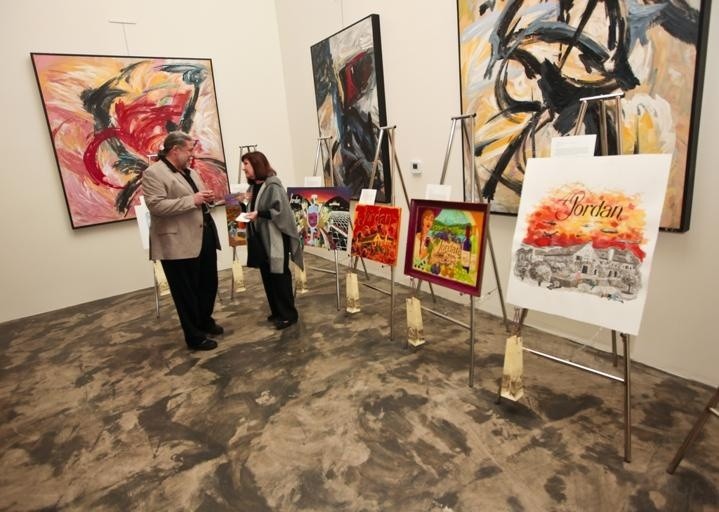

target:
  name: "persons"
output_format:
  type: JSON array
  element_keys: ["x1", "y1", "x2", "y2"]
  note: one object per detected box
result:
[
  {"x1": 140, "y1": 131, "x2": 224, "y2": 352},
  {"x1": 234, "y1": 151, "x2": 309, "y2": 336},
  {"x1": 410, "y1": 209, "x2": 435, "y2": 272}
]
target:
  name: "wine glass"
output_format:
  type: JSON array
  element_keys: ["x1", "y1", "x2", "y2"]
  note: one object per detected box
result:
[{"x1": 306, "y1": 206, "x2": 319, "y2": 245}]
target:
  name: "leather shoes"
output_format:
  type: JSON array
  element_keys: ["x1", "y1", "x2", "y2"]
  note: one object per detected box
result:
[
  {"x1": 187, "y1": 338, "x2": 218, "y2": 350},
  {"x1": 207, "y1": 322, "x2": 224, "y2": 334},
  {"x1": 267, "y1": 314, "x2": 298, "y2": 329}
]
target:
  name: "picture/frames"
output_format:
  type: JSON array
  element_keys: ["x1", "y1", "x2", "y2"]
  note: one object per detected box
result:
[
  {"x1": 30, "y1": 52, "x2": 230, "y2": 230},
  {"x1": 455, "y1": 0, "x2": 713, "y2": 233},
  {"x1": 404, "y1": 199, "x2": 491, "y2": 298},
  {"x1": 309, "y1": 14, "x2": 391, "y2": 204}
]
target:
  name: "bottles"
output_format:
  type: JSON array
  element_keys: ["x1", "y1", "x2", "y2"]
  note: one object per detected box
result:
[
  {"x1": 230, "y1": 224, "x2": 236, "y2": 237},
  {"x1": 470, "y1": 225, "x2": 477, "y2": 269},
  {"x1": 461, "y1": 225, "x2": 470, "y2": 271}
]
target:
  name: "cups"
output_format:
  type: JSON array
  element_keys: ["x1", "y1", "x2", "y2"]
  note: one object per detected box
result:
[{"x1": 237, "y1": 229, "x2": 247, "y2": 238}]
